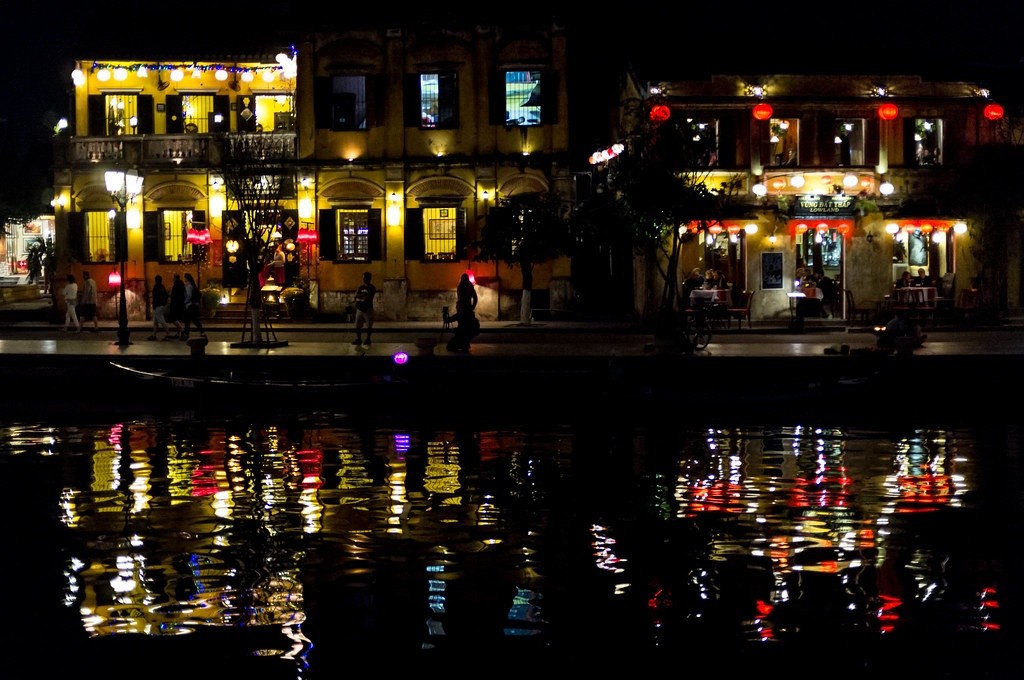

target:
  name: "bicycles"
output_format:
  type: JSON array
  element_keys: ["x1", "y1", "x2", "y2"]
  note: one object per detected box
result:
[{"x1": 683, "y1": 302, "x2": 722, "y2": 356}]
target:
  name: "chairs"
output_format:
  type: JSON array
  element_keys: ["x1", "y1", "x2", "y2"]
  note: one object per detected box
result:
[
  {"x1": 845, "y1": 288, "x2": 871, "y2": 327},
  {"x1": 912, "y1": 290, "x2": 937, "y2": 328},
  {"x1": 934, "y1": 273, "x2": 954, "y2": 308},
  {"x1": 955, "y1": 288, "x2": 979, "y2": 326},
  {"x1": 891, "y1": 289, "x2": 910, "y2": 323},
  {"x1": 728, "y1": 290, "x2": 756, "y2": 329}
]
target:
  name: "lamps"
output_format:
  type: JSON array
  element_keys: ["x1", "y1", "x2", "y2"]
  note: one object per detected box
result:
[
  {"x1": 391, "y1": 193, "x2": 397, "y2": 200},
  {"x1": 834, "y1": 124, "x2": 843, "y2": 144},
  {"x1": 866, "y1": 230, "x2": 874, "y2": 243},
  {"x1": 129, "y1": 116, "x2": 140, "y2": 129},
  {"x1": 483, "y1": 190, "x2": 488, "y2": 198},
  {"x1": 94, "y1": 68, "x2": 111, "y2": 84},
  {"x1": 58, "y1": 194, "x2": 67, "y2": 208},
  {"x1": 769, "y1": 119, "x2": 780, "y2": 144},
  {"x1": 299, "y1": 177, "x2": 311, "y2": 189},
  {"x1": 780, "y1": 120, "x2": 790, "y2": 129},
  {"x1": 210, "y1": 180, "x2": 220, "y2": 190},
  {"x1": 764, "y1": 222, "x2": 780, "y2": 245},
  {"x1": 843, "y1": 119, "x2": 854, "y2": 132}
]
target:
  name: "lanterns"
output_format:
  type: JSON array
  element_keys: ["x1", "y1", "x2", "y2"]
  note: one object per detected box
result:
[
  {"x1": 750, "y1": 105, "x2": 775, "y2": 120},
  {"x1": 649, "y1": 103, "x2": 673, "y2": 121},
  {"x1": 875, "y1": 101, "x2": 900, "y2": 122},
  {"x1": 983, "y1": 102, "x2": 1005, "y2": 121}
]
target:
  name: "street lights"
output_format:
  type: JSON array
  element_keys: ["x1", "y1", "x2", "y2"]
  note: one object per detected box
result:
[{"x1": 105, "y1": 163, "x2": 145, "y2": 347}]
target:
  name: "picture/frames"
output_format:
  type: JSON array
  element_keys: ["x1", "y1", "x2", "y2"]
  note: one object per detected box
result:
[
  {"x1": 231, "y1": 103, "x2": 237, "y2": 111},
  {"x1": 760, "y1": 250, "x2": 786, "y2": 292},
  {"x1": 156, "y1": 104, "x2": 165, "y2": 112}
]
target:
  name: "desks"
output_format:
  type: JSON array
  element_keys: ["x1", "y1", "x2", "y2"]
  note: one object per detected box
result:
[
  {"x1": 794, "y1": 288, "x2": 823, "y2": 299},
  {"x1": 892, "y1": 285, "x2": 938, "y2": 305},
  {"x1": 689, "y1": 288, "x2": 731, "y2": 311}
]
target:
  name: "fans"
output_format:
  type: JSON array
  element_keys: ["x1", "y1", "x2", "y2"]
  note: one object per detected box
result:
[
  {"x1": 227, "y1": 62, "x2": 241, "y2": 92},
  {"x1": 156, "y1": 61, "x2": 170, "y2": 92}
]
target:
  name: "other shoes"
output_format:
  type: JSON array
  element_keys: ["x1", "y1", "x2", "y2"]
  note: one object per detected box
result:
[
  {"x1": 146, "y1": 335, "x2": 156, "y2": 341},
  {"x1": 161, "y1": 337, "x2": 170, "y2": 341},
  {"x1": 364, "y1": 337, "x2": 372, "y2": 345},
  {"x1": 200, "y1": 334, "x2": 207, "y2": 338},
  {"x1": 352, "y1": 338, "x2": 363, "y2": 345},
  {"x1": 179, "y1": 335, "x2": 189, "y2": 341},
  {"x1": 170, "y1": 334, "x2": 180, "y2": 339},
  {"x1": 59, "y1": 328, "x2": 67, "y2": 333}
]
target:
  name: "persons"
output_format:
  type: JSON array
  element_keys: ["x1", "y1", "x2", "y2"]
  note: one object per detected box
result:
[
  {"x1": 351, "y1": 272, "x2": 375, "y2": 344},
  {"x1": 894, "y1": 271, "x2": 913, "y2": 286},
  {"x1": 58, "y1": 274, "x2": 81, "y2": 335},
  {"x1": 182, "y1": 273, "x2": 207, "y2": 338},
  {"x1": 442, "y1": 302, "x2": 479, "y2": 351},
  {"x1": 77, "y1": 271, "x2": 99, "y2": 333},
  {"x1": 688, "y1": 264, "x2": 745, "y2": 318},
  {"x1": 165, "y1": 273, "x2": 186, "y2": 337},
  {"x1": 146, "y1": 275, "x2": 170, "y2": 341},
  {"x1": 922, "y1": 149, "x2": 935, "y2": 165},
  {"x1": 456, "y1": 273, "x2": 478, "y2": 311},
  {"x1": 792, "y1": 257, "x2": 839, "y2": 318},
  {"x1": 915, "y1": 267, "x2": 932, "y2": 284}
]
